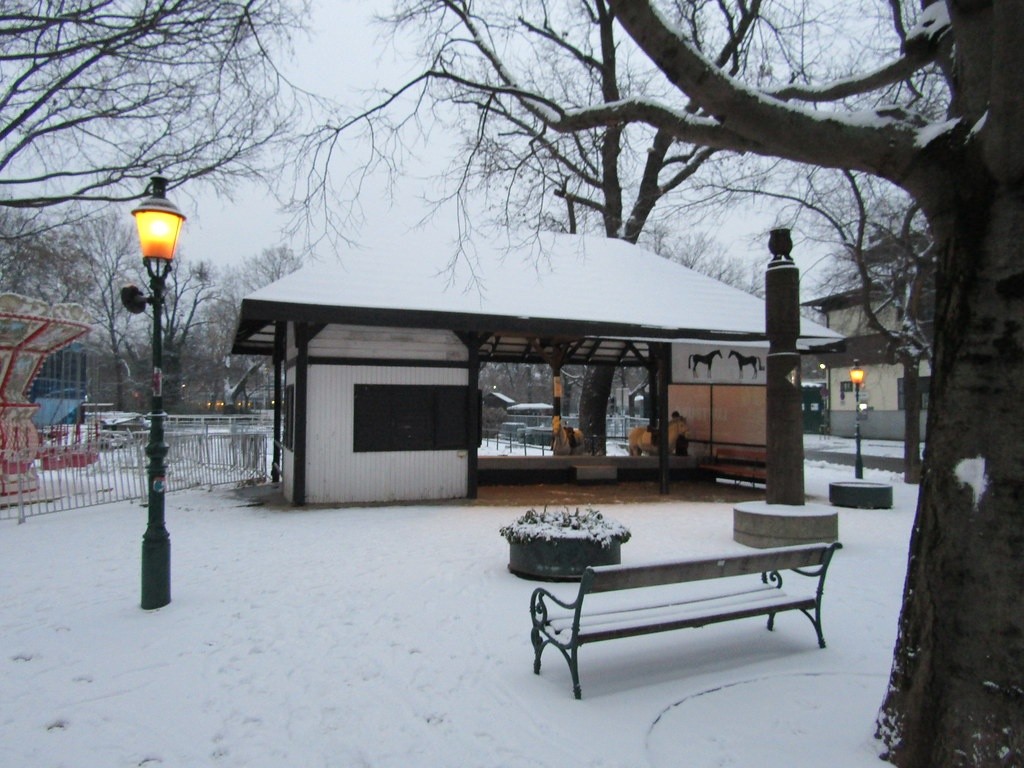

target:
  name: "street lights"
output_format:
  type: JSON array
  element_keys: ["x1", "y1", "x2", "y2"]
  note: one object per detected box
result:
[
  {"x1": 848, "y1": 359, "x2": 873, "y2": 481},
  {"x1": 118, "y1": 161, "x2": 186, "y2": 609}
]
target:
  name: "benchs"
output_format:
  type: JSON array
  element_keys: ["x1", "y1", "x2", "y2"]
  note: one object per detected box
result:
[{"x1": 528, "y1": 542, "x2": 844, "y2": 700}]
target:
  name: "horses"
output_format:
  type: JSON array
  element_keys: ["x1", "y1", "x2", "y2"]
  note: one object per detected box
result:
[
  {"x1": 551, "y1": 415, "x2": 584, "y2": 456},
  {"x1": 629, "y1": 416, "x2": 691, "y2": 457}
]
